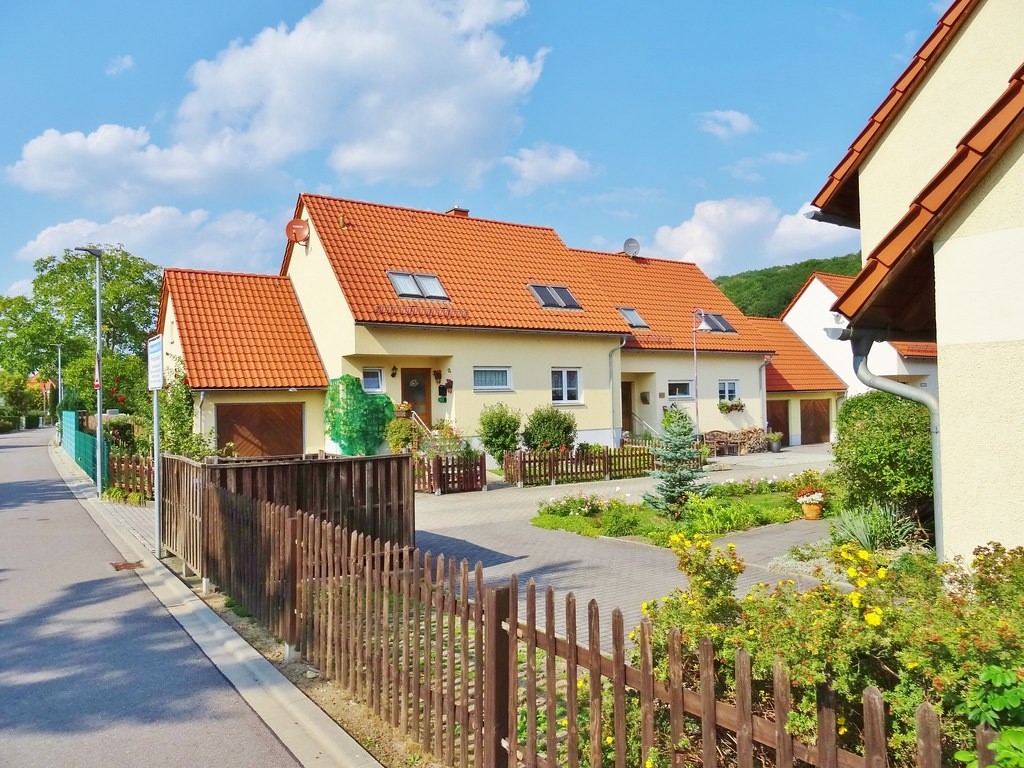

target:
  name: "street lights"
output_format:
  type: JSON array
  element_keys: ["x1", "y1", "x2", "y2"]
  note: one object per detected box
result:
[
  {"x1": 692, "y1": 307, "x2": 713, "y2": 443},
  {"x1": 49, "y1": 343, "x2": 64, "y2": 448},
  {"x1": 74, "y1": 247, "x2": 103, "y2": 499}
]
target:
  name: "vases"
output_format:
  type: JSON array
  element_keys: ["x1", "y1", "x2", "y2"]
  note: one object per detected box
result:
[
  {"x1": 448, "y1": 388, "x2": 452, "y2": 393},
  {"x1": 393, "y1": 410, "x2": 411, "y2": 417},
  {"x1": 802, "y1": 503, "x2": 822, "y2": 520},
  {"x1": 436, "y1": 378, "x2": 440, "y2": 383}
]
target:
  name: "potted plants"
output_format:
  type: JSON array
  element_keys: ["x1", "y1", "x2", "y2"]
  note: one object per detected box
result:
[
  {"x1": 766, "y1": 432, "x2": 784, "y2": 453},
  {"x1": 717, "y1": 401, "x2": 731, "y2": 414},
  {"x1": 732, "y1": 399, "x2": 746, "y2": 412}
]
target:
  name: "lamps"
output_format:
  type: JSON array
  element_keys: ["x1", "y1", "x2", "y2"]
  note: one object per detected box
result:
[{"x1": 391, "y1": 365, "x2": 398, "y2": 377}]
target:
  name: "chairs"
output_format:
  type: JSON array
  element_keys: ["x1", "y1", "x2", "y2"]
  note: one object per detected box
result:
[{"x1": 703, "y1": 430, "x2": 741, "y2": 458}]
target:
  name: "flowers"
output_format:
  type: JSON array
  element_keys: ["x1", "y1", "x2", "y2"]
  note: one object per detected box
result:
[
  {"x1": 795, "y1": 485, "x2": 832, "y2": 504},
  {"x1": 446, "y1": 379, "x2": 454, "y2": 388},
  {"x1": 433, "y1": 369, "x2": 441, "y2": 379},
  {"x1": 394, "y1": 401, "x2": 412, "y2": 410}
]
